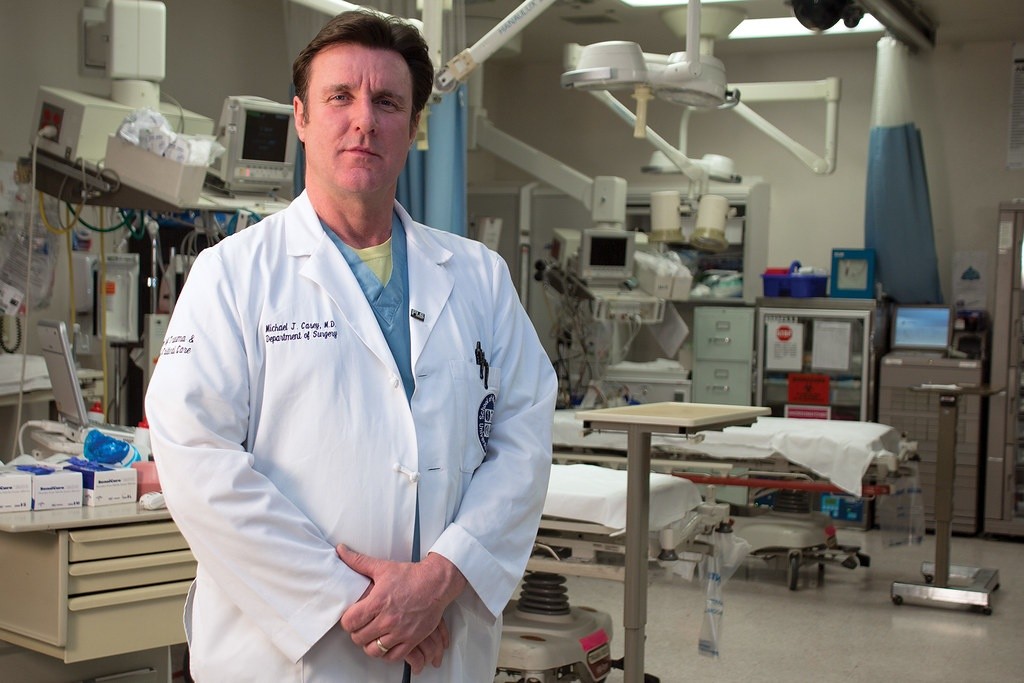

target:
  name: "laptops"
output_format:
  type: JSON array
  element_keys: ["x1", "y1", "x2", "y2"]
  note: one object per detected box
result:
[
  {"x1": 34, "y1": 318, "x2": 138, "y2": 440},
  {"x1": 884, "y1": 304, "x2": 954, "y2": 361}
]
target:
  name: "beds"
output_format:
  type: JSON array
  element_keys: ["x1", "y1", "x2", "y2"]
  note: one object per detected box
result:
[
  {"x1": 524, "y1": 463, "x2": 730, "y2": 586},
  {"x1": 553, "y1": 408, "x2": 920, "y2": 590}
]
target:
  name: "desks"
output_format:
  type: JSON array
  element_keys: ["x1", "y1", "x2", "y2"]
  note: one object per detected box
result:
[
  {"x1": 888, "y1": 382, "x2": 1006, "y2": 615},
  {"x1": 573, "y1": 398, "x2": 770, "y2": 683}
]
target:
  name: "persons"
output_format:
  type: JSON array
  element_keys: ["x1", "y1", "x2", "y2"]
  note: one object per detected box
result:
[{"x1": 145, "y1": 12, "x2": 559, "y2": 683}]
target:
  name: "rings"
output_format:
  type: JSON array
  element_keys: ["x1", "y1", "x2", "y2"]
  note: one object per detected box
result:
[{"x1": 375, "y1": 637, "x2": 389, "y2": 653}]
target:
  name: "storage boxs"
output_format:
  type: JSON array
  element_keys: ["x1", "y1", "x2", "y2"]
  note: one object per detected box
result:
[
  {"x1": 0, "y1": 457, "x2": 137, "y2": 514},
  {"x1": 761, "y1": 261, "x2": 831, "y2": 298},
  {"x1": 103, "y1": 134, "x2": 205, "y2": 209}
]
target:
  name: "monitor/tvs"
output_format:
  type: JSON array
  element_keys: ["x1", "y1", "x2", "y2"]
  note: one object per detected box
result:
[
  {"x1": 579, "y1": 229, "x2": 635, "y2": 280},
  {"x1": 209, "y1": 96, "x2": 298, "y2": 181}
]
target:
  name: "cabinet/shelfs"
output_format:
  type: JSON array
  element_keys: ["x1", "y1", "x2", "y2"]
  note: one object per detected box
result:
[{"x1": 690, "y1": 304, "x2": 756, "y2": 404}]
